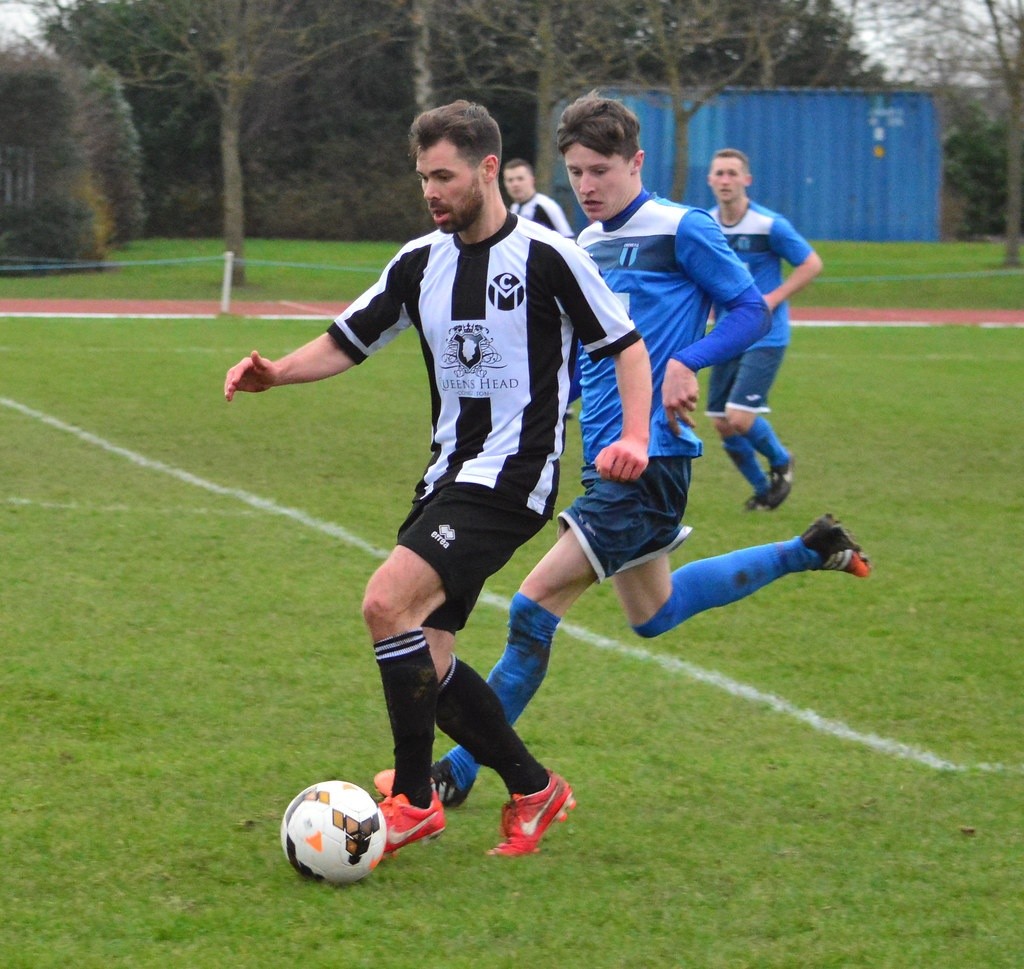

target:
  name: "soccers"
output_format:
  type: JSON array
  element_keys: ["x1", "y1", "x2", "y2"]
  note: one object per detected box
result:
[{"x1": 280, "y1": 779, "x2": 386, "y2": 885}]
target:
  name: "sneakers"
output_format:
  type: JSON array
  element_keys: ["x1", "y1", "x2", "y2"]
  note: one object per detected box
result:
[
  {"x1": 377, "y1": 777, "x2": 446, "y2": 853},
  {"x1": 766, "y1": 452, "x2": 795, "y2": 508},
  {"x1": 373, "y1": 759, "x2": 477, "y2": 808},
  {"x1": 746, "y1": 493, "x2": 773, "y2": 510},
  {"x1": 800, "y1": 513, "x2": 872, "y2": 577},
  {"x1": 485, "y1": 768, "x2": 577, "y2": 857}
]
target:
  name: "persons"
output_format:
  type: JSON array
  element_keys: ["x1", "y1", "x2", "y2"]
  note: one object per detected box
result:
[
  {"x1": 502, "y1": 159, "x2": 576, "y2": 239},
  {"x1": 220, "y1": 99, "x2": 651, "y2": 856},
  {"x1": 703, "y1": 148, "x2": 825, "y2": 511},
  {"x1": 374, "y1": 90, "x2": 869, "y2": 806}
]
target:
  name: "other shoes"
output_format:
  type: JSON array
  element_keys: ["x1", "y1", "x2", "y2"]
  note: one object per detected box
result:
[{"x1": 566, "y1": 406, "x2": 574, "y2": 418}]
